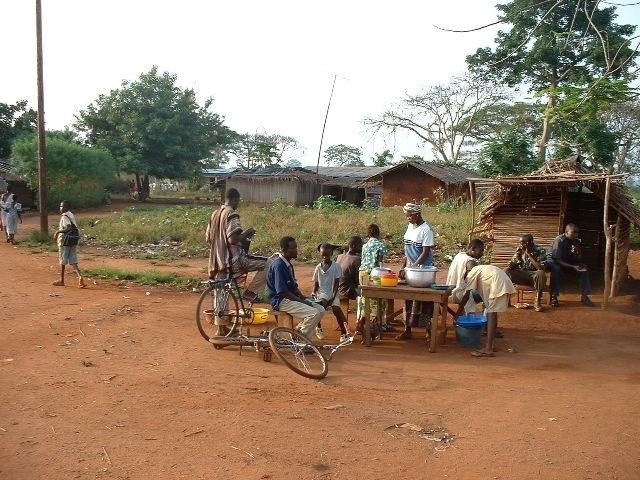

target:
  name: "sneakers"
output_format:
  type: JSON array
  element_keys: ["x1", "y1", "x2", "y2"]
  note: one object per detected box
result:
[
  {"x1": 382, "y1": 324, "x2": 392, "y2": 332},
  {"x1": 316, "y1": 327, "x2": 324, "y2": 340},
  {"x1": 340, "y1": 334, "x2": 350, "y2": 343},
  {"x1": 534, "y1": 302, "x2": 543, "y2": 312}
]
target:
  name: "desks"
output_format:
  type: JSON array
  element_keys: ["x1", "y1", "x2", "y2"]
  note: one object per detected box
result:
[{"x1": 357, "y1": 283, "x2": 449, "y2": 354}]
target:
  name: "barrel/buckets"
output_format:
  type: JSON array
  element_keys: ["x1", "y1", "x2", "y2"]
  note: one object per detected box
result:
[{"x1": 456, "y1": 325, "x2": 482, "y2": 346}]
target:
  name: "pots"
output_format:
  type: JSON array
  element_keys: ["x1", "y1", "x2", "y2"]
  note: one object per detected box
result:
[{"x1": 402, "y1": 263, "x2": 437, "y2": 287}]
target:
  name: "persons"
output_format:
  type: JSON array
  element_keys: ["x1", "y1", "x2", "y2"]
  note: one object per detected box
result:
[
  {"x1": 0, "y1": 185, "x2": 12, "y2": 236},
  {"x1": 454, "y1": 261, "x2": 517, "y2": 357},
  {"x1": 310, "y1": 244, "x2": 353, "y2": 344},
  {"x1": 0, "y1": 193, "x2": 22, "y2": 243},
  {"x1": 354, "y1": 223, "x2": 385, "y2": 338},
  {"x1": 335, "y1": 236, "x2": 395, "y2": 332},
  {"x1": 394, "y1": 203, "x2": 436, "y2": 340},
  {"x1": 51, "y1": 200, "x2": 85, "y2": 288},
  {"x1": 546, "y1": 223, "x2": 594, "y2": 306},
  {"x1": 502, "y1": 234, "x2": 548, "y2": 311},
  {"x1": 130, "y1": 179, "x2": 136, "y2": 200},
  {"x1": 206, "y1": 188, "x2": 268, "y2": 337},
  {"x1": 265, "y1": 235, "x2": 325, "y2": 355},
  {"x1": 447, "y1": 239, "x2": 505, "y2": 337}
]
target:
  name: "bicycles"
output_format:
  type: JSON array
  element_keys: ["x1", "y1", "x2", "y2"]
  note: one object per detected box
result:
[
  {"x1": 196, "y1": 255, "x2": 270, "y2": 342},
  {"x1": 208, "y1": 326, "x2": 358, "y2": 380}
]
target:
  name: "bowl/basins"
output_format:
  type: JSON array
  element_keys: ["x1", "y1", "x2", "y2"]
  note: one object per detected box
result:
[
  {"x1": 242, "y1": 308, "x2": 270, "y2": 325},
  {"x1": 374, "y1": 274, "x2": 399, "y2": 286},
  {"x1": 457, "y1": 315, "x2": 488, "y2": 329},
  {"x1": 370, "y1": 267, "x2": 392, "y2": 279},
  {"x1": 203, "y1": 308, "x2": 234, "y2": 325}
]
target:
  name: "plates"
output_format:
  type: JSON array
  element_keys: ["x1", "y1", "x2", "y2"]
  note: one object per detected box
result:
[{"x1": 431, "y1": 284, "x2": 450, "y2": 289}]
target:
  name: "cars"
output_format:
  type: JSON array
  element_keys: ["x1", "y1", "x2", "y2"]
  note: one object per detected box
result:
[{"x1": 148, "y1": 181, "x2": 174, "y2": 192}]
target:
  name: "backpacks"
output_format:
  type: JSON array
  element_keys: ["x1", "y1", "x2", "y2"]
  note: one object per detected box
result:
[{"x1": 61, "y1": 215, "x2": 81, "y2": 246}]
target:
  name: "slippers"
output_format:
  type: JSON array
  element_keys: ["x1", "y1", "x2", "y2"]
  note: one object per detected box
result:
[
  {"x1": 395, "y1": 331, "x2": 413, "y2": 341},
  {"x1": 80, "y1": 284, "x2": 87, "y2": 288},
  {"x1": 471, "y1": 349, "x2": 495, "y2": 357},
  {"x1": 424, "y1": 333, "x2": 431, "y2": 342},
  {"x1": 243, "y1": 294, "x2": 263, "y2": 302},
  {"x1": 53, "y1": 281, "x2": 65, "y2": 286}
]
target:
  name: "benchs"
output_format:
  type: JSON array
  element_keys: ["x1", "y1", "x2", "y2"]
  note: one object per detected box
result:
[
  {"x1": 384, "y1": 295, "x2": 465, "y2": 330},
  {"x1": 506, "y1": 270, "x2": 554, "y2": 308},
  {"x1": 268, "y1": 293, "x2": 351, "y2": 343}
]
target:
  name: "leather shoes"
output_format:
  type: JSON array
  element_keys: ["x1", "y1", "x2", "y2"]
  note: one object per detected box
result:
[
  {"x1": 550, "y1": 294, "x2": 559, "y2": 307},
  {"x1": 581, "y1": 295, "x2": 593, "y2": 306}
]
